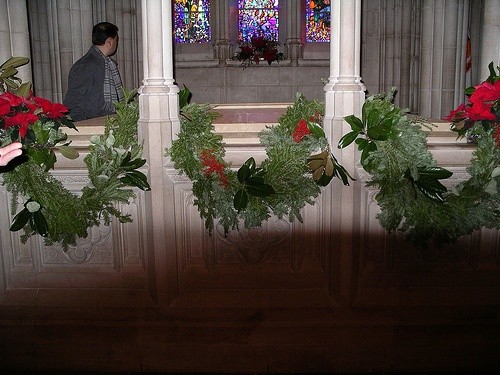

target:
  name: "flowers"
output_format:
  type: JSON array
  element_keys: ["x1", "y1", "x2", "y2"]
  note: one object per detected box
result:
[
  {"x1": 234, "y1": 37, "x2": 286, "y2": 70},
  {"x1": 0, "y1": 61, "x2": 500, "y2": 252}
]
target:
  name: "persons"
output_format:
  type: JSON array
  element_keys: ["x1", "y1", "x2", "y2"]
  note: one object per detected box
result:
[{"x1": 65, "y1": 22, "x2": 126, "y2": 122}]
